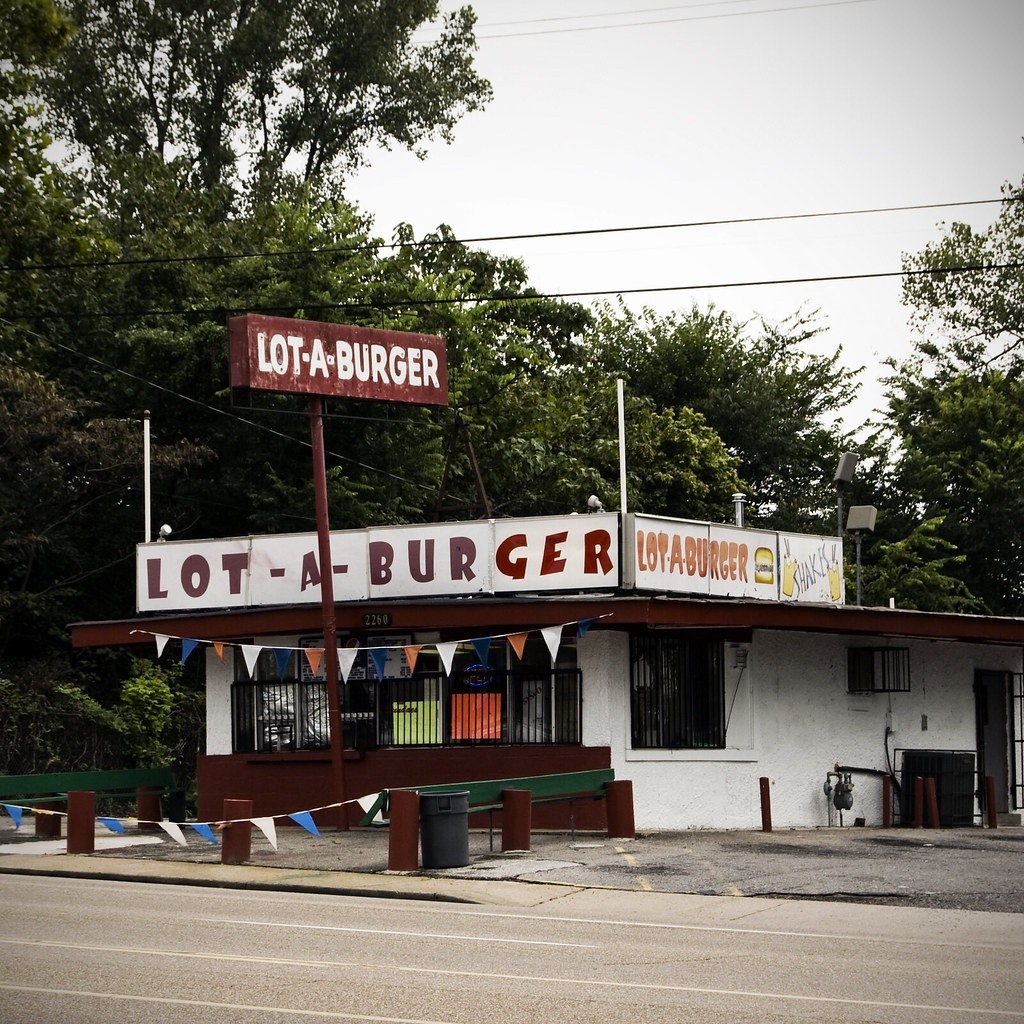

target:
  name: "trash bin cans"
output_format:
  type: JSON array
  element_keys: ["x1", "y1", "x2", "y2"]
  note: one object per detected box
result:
[
  {"x1": 419, "y1": 789, "x2": 472, "y2": 871},
  {"x1": 901, "y1": 751, "x2": 976, "y2": 827}
]
